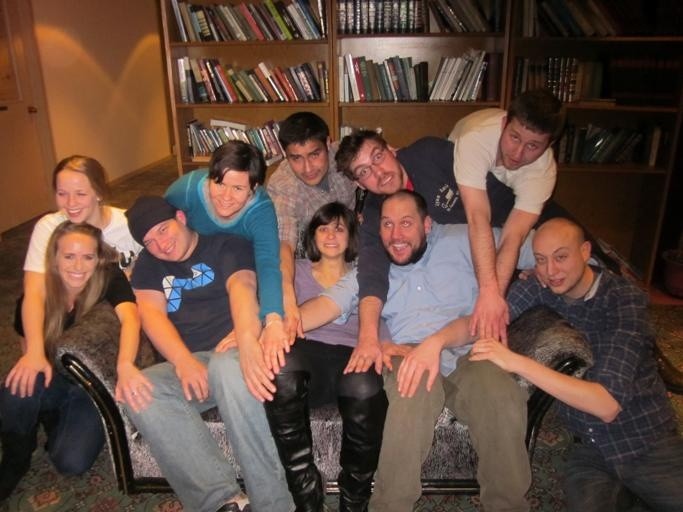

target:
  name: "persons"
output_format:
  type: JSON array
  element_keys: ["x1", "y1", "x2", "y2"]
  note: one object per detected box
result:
[
  {"x1": 162, "y1": 140, "x2": 289, "y2": 373},
  {"x1": 396, "y1": 218, "x2": 682, "y2": 512},
  {"x1": 334, "y1": 129, "x2": 682, "y2": 396},
  {"x1": 447, "y1": 90, "x2": 559, "y2": 347},
  {"x1": 123, "y1": 196, "x2": 295, "y2": 511},
  {"x1": 217, "y1": 190, "x2": 604, "y2": 511},
  {"x1": 6, "y1": 155, "x2": 142, "y2": 397},
  {"x1": 215, "y1": 202, "x2": 412, "y2": 511},
  {"x1": 268, "y1": 112, "x2": 359, "y2": 346},
  {"x1": 0, "y1": 219, "x2": 153, "y2": 503}
]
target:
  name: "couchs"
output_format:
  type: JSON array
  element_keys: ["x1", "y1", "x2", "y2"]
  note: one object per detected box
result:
[{"x1": 55, "y1": 297, "x2": 594, "y2": 493}]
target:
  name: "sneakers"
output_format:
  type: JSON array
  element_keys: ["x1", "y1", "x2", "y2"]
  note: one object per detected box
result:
[{"x1": 0, "y1": 461, "x2": 30, "y2": 500}]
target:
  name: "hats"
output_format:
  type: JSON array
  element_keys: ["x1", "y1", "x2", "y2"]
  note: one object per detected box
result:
[{"x1": 124, "y1": 195, "x2": 180, "y2": 247}]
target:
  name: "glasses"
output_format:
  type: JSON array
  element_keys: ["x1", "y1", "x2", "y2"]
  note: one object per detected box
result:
[{"x1": 352, "y1": 149, "x2": 386, "y2": 180}]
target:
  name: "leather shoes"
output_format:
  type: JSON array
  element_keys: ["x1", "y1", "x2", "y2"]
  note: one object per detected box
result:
[
  {"x1": 660, "y1": 356, "x2": 683, "y2": 394},
  {"x1": 215, "y1": 502, "x2": 252, "y2": 512}
]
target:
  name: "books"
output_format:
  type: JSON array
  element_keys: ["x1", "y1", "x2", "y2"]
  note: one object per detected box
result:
[
  {"x1": 556, "y1": 110, "x2": 672, "y2": 168},
  {"x1": 338, "y1": 1, "x2": 507, "y2": 35},
  {"x1": 523, "y1": 0, "x2": 619, "y2": 38},
  {"x1": 177, "y1": 55, "x2": 327, "y2": 103},
  {"x1": 338, "y1": 47, "x2": 500, "y2": 102},
  {"x1": 185, "y1": 118, "x2": 286, "y2": 160},
  {"x1": 597, "y1": 235, "x2": 638, "y2": 278},
  {"x1": 173, "y1": 0, "x2": 327, "y2": 43},
  {"x1": 515, "y1": 54, "x2": 683, "y2": 106},
  {"x1": 339, "y1": 124, "x2": 382, "y2": 140}
]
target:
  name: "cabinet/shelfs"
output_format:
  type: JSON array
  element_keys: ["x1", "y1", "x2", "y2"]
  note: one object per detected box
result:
[
  {"x1": 156, "y1": 1, "x2": 332, "y2": 175},
  {"x1": 505, "y1": 2, "x2": 683, "y2": 288},
  {"x1": 330, "y1": 1, "x2": 504, "y2": 144}
]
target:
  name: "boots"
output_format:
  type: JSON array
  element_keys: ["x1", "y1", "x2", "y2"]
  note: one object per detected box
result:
[
  {"x1": 263, "y1": 369, "x2": 324, "y2": 512},
  {"x1": 337, "y1": 390, "x2": 389, "y2": 512}
]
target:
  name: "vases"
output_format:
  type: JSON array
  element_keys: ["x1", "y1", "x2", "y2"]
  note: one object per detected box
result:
[{"x1": 663, "y1": 249, "x2": 683, "y2": 298}]
